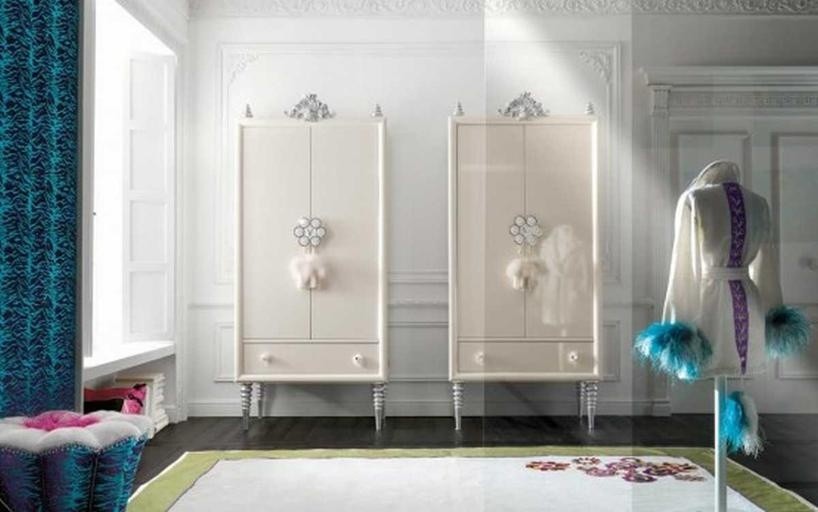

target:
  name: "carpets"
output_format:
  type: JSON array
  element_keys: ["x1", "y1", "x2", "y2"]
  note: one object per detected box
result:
[{"x1": 119, "y1": 446, "x2": 818, "y2": 511}]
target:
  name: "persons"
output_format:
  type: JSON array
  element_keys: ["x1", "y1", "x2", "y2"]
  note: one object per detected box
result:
[
  {"x1": 533, "y1": 223, "x2": 591, "y2": 332},
  {"x1": 629, "y1": 158, "x2": 811, "y2": 381}
]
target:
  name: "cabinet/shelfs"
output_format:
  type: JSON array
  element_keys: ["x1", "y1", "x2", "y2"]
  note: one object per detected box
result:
[
  {"x1": 235, "y1": 117, "x2": 388, "y2": 431},
  {"x1": 446, "y1": 115, "x2": 601, "y2": 430}
]
